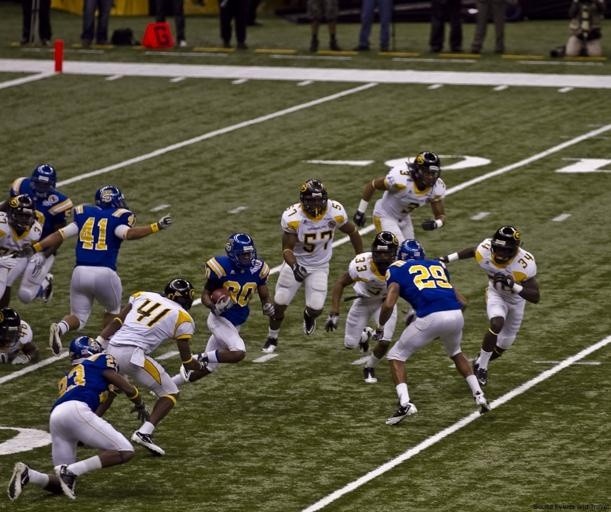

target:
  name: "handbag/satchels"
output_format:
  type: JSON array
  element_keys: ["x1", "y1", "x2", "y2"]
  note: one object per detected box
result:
[{"x1": 111, "y1": 28, "x2": 135, "y2": 47}]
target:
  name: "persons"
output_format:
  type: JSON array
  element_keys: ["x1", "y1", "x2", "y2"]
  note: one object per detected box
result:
[
  {"x1": 21, "y1": 183, "x2": 174, "y2": 353},
  {"x1": 372, "y1": 239, "x2": 492, "y2": 427},
  {"x1": 77, "y1": 276, "x2": 215, "y2": 458},
  {"x1": 16, "y1": 1, "x2": 55, "y2": 49},
  {"x1": 304, "y1": 0, "x2": 344, "y2": 53},
  {"x1": 434, "y1": 224, "x2": 543, "y2": 385},
  {"x1": 354, "y1": 152, "x2": 449, "y2": 255},
  {"x1": 1, "y1": 195, "x2": 46, "y2": 301},
  {"x1": 429, "y1": 1, "x2": 466, "y2": 56},
  {"x1": 80, "y1": 0, "x2": 114, "y2": 50},
  {"x1": 565, "y1": 0, "x2": 609, "y2": 58},
  {"x1": 217, "y1": 0, "x2": 250, "y2": 51},
  {"x1": 324, "y1": 233, "x2": 403, "y2": 382},
  {"x1": 7, "y1": 335, "x2": 152, "y2": 502},
  {"x1": 0, "y1": 305, "x2": 40, "y2": 367},
  {"x1": 155, "y1": 0, "x2": 188, "y2": 49},
  {"x1": 469, "y1": 0, "x2": 514, "y2": 55},
  {"x1": 351, "y1": 0, "x2": 397, "y2": 54},
  {"x1": 171, "y1": 233, "x2": 276, "y2": 386},
  {"x1": 1, "y1": 165, "x2": 75, "y2": 309},
  {"x1": 260, "y1": 180, "x2": 366, "y2": 354}
]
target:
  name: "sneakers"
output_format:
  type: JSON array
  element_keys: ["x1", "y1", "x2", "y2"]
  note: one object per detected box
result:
[
  {"x1": 356, "y1": 326, "x2": 371, "y2": 352},
  {"x1": 7, "y1": 461, "x2": 34, "y2": 502},
  {"x1": 131, "y1": 428, "x2": 166, "y2": 456},
  {"x1": 260, "y1": 338, "x2": 278, "y2": 353},
  {"x1": 473, "y1": 360, "x2": 488, "y2": 388},
  {"x1": 302, "y1": 308, "x2": 316, "y2": 335},
  {"x1": 39, "y1": 275, "x2": 55, "y2": 302},
  {"x1": 475, "y1": 390, "x2": 491, "y2": 415},
  {"x1": 54, "y1": 463, "x2": 78, "y2": 501},
  {"x1": 180, "y1": 352, "x2": 199, "y2": 380},
  {"x1": 385, "y1": 401, "x2": 417, "y2": 425},
  {"x1": 49, "y1": 323, "x2": 63, "y2": 358},
  {"x1": 363, "y1": 362, "x2": 377, "y2": 386}
]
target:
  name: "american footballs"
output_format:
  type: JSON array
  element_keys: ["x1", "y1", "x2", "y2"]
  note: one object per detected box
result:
[{"x1": 210, "y1": 288, "x2": 230, "y2": 303}]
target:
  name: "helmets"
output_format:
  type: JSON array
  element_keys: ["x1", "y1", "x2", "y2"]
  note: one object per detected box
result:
[
  {"x1": 300, "y1": 179, "x2": 327, "y2": 218},
  {"x1": 397, "y1": 240, "x2": 427, "y2": 261},
  {"x1": 68, "y1": 336, "x2": 101, "y2": 365},
  {"x1": 491, "y1": 225, "x2": 521, "y2": 263},
  {"x1": 371, "y1": 230, "x2": 398, "y2": 276},
  {"x1": 162, "y1": 278, "x2": 196, "y2": 312},
  {"x1": 411, "y1": 151, "x2": 441, "y2": 190},
  {"x1": 0, "y1": 307, "x2": 20, "y2": 349},
  {"x1": 95, "y1": 186, "x2": 129, "y2": 210},
  {"x1": 7, "y1": 195, "x2": 38, "y2": 239},
  {"x1": 225, "y1": 233, "x2": 256, "y2": 273},
  {"x1": 30, "y1": 165, "x2": 57, "y2": 197}
]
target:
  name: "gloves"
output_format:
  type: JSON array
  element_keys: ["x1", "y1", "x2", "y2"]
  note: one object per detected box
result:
[
  {"x1": 325, "y1": 312, "x2": 340, "y2": 332},
  {"x1": 12, "y1": 354, "x2": 32, "y2": 364},
  {"x1": 14, "y1": 243, "x2": 41, "y2": 259},
  {"x1": 130, "y1": 403, "x2": 150, "y2": 425},
  {"x1": 293, "y1": 263, "x2": 310, "y2": 281},
  {"x1": 371, "y1": 328, "x2": 384, "y2": 340},
  {"x1": 199, "y1": 355, "x2": 209, "y2": 371},
  {"x1": 263, "y1": 302, "x2": 275, "y2": 315},
  {"x1": 421, "y1": 218, "x2": 439, "y2": 232},
  {"x1": 493, "y1": 272, "x2": 523, "y2": 294},
  {"x1": 353, "y1": 211, "x2": 366, "y2": 225},
  {"x1": 27, "y1": 253, "x2": 46, "y2": 276},
  {"x1": 0, "y1": 255, "x2": 16, "y2": 268},
  {"x1": 151, "y1": 212, "x2": 173, "y2": 233},
  {"x1": 216, "y1": 297, "x2": 232, "y2": 314}
]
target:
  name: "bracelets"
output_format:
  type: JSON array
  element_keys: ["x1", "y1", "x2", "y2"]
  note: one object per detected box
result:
[
  {"x1": 511, "y1": 282, "x2": 527, "y2": 295},
  {"x1": 434, "y1": 218, "x2": 445, "y2": 229},
  {"x1": 149, "y1": 222, "x2": 160, "y2": 233},
  {"x1": 358, "y1": 198, "x2": 369, "y2": 215},
  {"x1": 446, "y1": 251, "x2": 462, "y2": 262},
  {"x1": 31, "y1": 240, "x2": 43, "y2": 254},
  {"x1": 134, "y1": 400, "x2": 147, "y2": 411}
]
